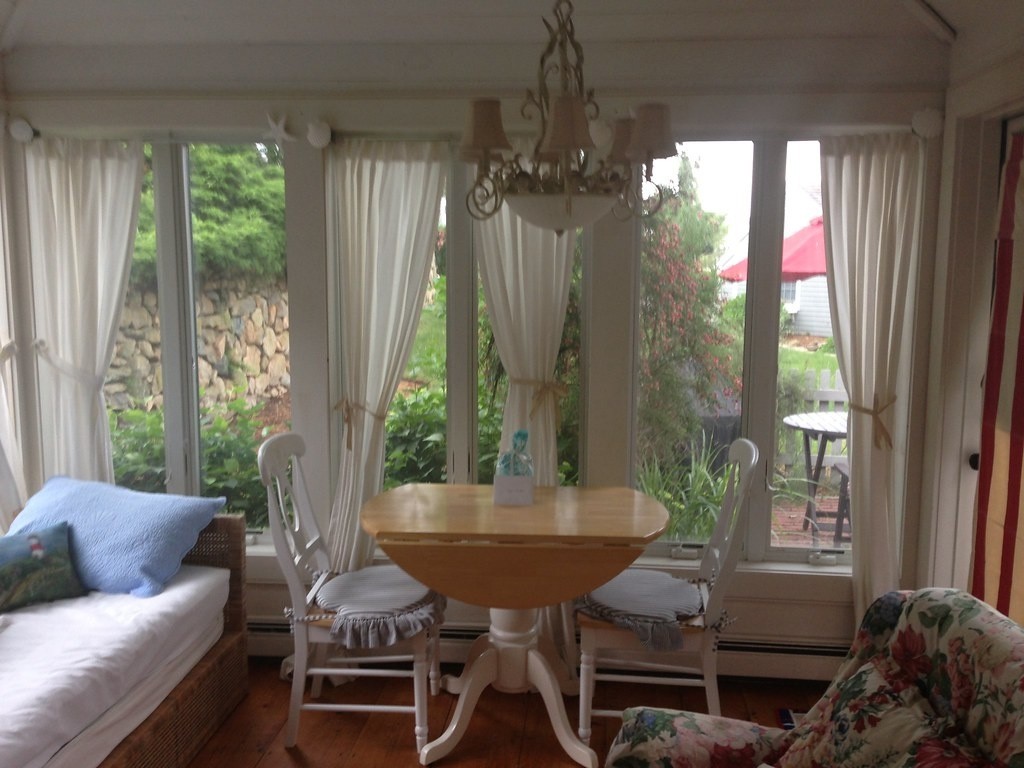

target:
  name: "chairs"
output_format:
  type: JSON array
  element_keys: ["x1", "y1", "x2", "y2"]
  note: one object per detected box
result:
[
  {"x1": 256, "y1": 432, "x2": 449, "y2": 755},
  {"x1": 570, "y1": 436, "x2": 760, "y2": 750}
]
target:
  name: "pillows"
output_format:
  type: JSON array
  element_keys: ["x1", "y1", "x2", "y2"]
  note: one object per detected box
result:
[
  {"x1": 0, "y1": 519, "x2": 89, "y2": 615},
  {"x1": 4, "y1": 476, "x2": 228, "y2": 598}
]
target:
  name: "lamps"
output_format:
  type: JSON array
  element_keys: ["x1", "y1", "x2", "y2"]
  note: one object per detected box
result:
[{"x1": 459, "y1": 0, "x2": 678, "y2": 240}]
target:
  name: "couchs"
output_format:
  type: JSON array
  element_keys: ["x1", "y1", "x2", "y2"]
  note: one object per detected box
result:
[
  {"x1": 0, "y1": 513, "x2": 252, "y2": 768},
  {"x1": 604, "y1": 588, "x2": 1024, "y2": 768}
]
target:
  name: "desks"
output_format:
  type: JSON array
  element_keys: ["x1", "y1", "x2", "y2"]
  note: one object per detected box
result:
[
  {"x1": 360, "y1": 482, "x2": 671, "y2": 768},
  {"x1": 783, "y1": 411, "x2": 851, "y2": 551}
]
